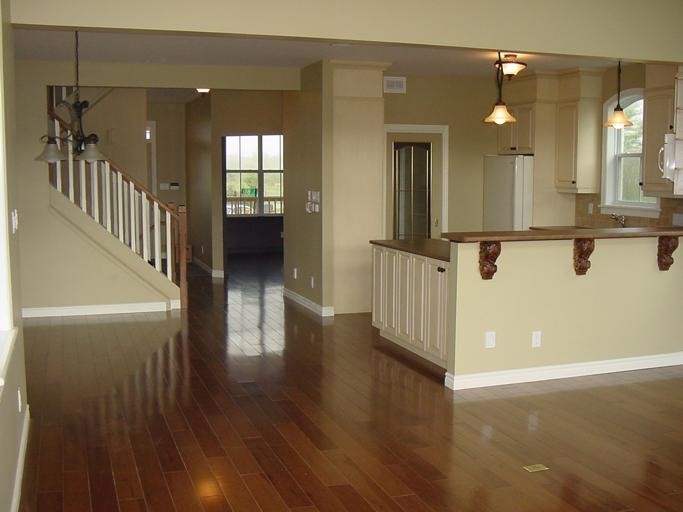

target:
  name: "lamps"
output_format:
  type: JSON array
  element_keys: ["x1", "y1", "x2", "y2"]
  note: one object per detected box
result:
[
  {"x1": 483, "y1": 52, "x2": 528, "y2": 125},
  {"x1": 603, "y1": 60, "x2": 634, "y2": 130},
  {"x1": 33, "y1": 32, "x2": 107, "y2": 164}
]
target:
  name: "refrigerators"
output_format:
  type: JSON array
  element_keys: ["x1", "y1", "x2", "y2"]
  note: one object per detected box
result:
[{"x1": 483, "y1": 154, "x2": 533, "y2": 231}]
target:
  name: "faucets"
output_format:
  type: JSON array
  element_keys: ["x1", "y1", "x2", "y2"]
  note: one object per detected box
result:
[{"x1": 608, "y1": 211, "x2": 628, "y2": 228}]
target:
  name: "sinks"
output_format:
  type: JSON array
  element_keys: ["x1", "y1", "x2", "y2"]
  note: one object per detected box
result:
[{"x1": 529, "y1": 225, "x2": 600, "y2": 230}]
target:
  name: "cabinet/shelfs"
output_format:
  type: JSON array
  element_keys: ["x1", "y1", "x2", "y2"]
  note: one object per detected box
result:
[
  {"x1": 371, "y1": 244, "x2": 448, "y2": 370},
  {"x1": 495, "y1": 102, "x2": 555, "y2": 156},
  {"x1": 554, "y1": 99, "x2": 602, "y2": 194},
  {"x1": 641, "y1": 86, "x2": 682, "y2": 199}
]
